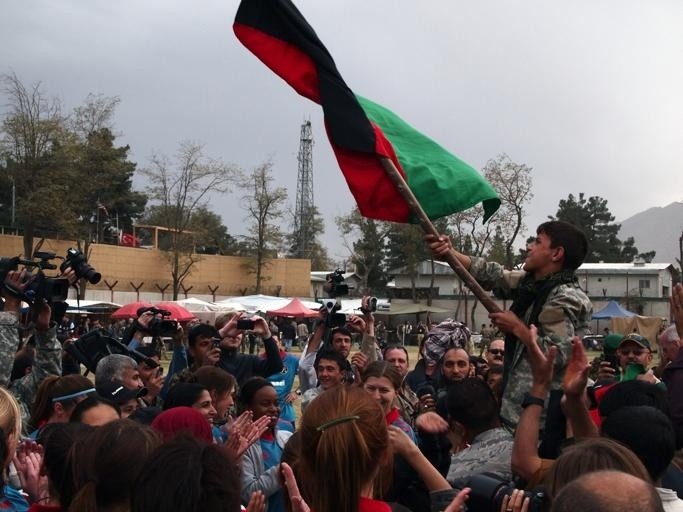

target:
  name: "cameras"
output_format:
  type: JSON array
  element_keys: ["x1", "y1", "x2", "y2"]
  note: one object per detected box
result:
[
  {"x1": 237, "y1": 319, "x2": 254, "y2": 331},
  {"x1": 465, "y1": 472, "x2": 547, "y2": 512},
  {"x1": 367, "y1": 296, "x2": 377, "y2": 313},
  {"x1": 60, "y1": 247, "x2": 100, "y2": 285}
]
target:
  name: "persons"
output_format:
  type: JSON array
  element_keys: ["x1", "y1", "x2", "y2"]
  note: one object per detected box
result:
[{"x1": 0, "y1": 221, "x2": 682, "y2": 512}]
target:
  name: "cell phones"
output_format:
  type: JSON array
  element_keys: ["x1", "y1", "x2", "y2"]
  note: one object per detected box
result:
[
  {"x1": 155, "y1": 368, "x2": 163, "y2": 378},
  {"x1": 602, "y1": 343, "x2": 621, "y2": 375},
  {"x1": 211, "y1": 338, "x2": 220, "y2": 350}
]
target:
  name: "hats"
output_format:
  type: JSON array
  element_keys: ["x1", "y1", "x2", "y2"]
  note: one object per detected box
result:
[{"x1": 616, "y1": 334, "x2": 650, "y2": 356}]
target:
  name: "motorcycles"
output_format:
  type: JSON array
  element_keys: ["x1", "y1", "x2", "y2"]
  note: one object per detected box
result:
[{"x1": 300, "y1": 332, "x2": 316, "y2": 352}]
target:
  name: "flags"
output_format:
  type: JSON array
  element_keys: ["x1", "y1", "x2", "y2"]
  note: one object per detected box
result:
[{"x1": 233, "y1": 0, "x2": 501, "y2": 225}]
